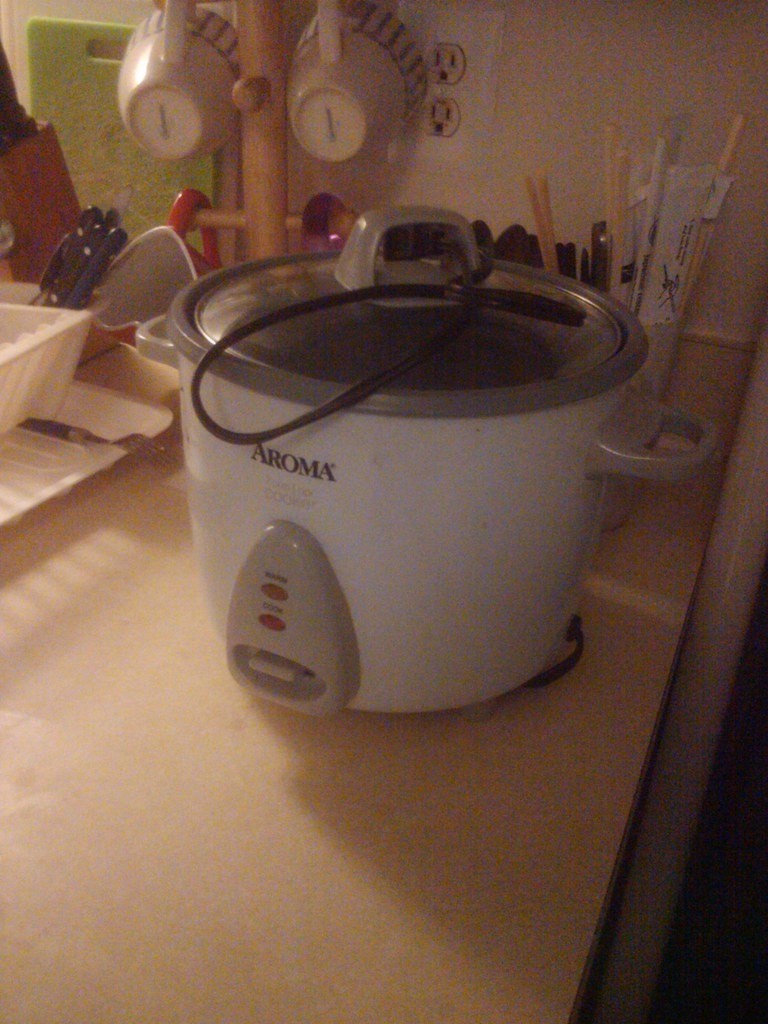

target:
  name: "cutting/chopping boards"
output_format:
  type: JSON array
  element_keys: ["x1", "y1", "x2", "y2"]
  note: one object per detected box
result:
[{"x1": 24, "y1": 16, "x2": 225, "y2": 256}]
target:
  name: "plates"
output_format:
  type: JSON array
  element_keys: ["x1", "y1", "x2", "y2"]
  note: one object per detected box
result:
[{"x1": 0, "y1": 380, "x2": 173, "y2": 533}]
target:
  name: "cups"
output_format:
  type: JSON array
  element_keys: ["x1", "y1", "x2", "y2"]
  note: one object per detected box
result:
[
  {"x1": 117, "y1": 0, "x2": 239, "y2": 164},
  {"x1": 301, "y1": 193, "x2": 349, "y2": 252},
  {"x1": 283, "y1": 0, "x2": 429, "y2": 165},
  {"x1": 135, "y1": 312, "x2": 180, "y2": 370},
  {"x1": 89, "y1": 188, "x2": 223, "y2": 344}
]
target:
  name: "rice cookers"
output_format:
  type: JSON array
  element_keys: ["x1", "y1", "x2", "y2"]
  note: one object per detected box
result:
[{"x1": 133, "y1": 206, "x2": 712, "y2": 718}]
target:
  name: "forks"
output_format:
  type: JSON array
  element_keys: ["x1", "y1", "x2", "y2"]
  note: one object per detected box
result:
[{"x1": 16, "y1": 416, "x2": 178, "y2": 472}]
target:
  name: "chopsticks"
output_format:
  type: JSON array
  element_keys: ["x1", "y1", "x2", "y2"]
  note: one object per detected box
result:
[
  {"x1": 524, "y1": 168, "x2": 560, "y2": 276},
  {"x1": 603, "y1": 122, "x2": 630, "y2": 303}
]
